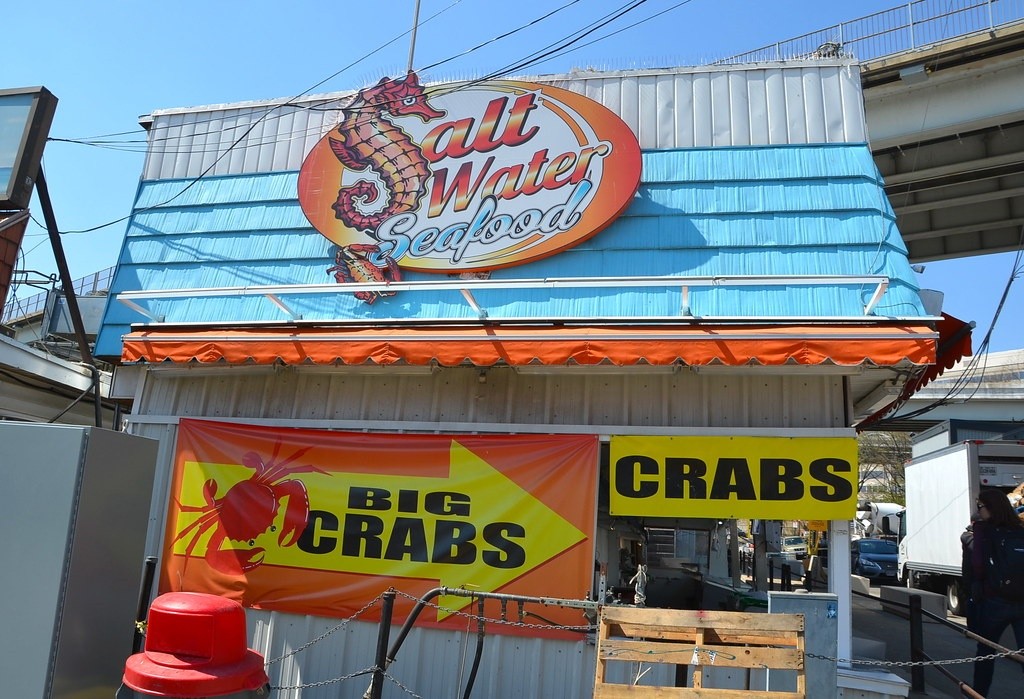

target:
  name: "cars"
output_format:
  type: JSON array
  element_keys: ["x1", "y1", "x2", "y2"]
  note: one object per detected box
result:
[
  {"x1": 780, "y1": 536, "x2": 807, "y2": 557},
  {"x1": 850, "y1": 538, "x2": 899, "y2": 584}
]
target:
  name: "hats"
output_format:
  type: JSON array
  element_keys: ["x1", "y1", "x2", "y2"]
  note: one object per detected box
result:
[{"x1": 971, "y1": 513, "x2": 983, "y2": 521}]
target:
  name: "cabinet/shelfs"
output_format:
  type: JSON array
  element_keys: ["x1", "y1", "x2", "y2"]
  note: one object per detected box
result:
[{"x1": 644, "y1": 526, "x2": 676, "y2": 566}]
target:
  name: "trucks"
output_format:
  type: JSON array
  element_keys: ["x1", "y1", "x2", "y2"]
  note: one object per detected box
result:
[
  {"x1": 882, "y1": 439, "x2": 1024, "y2": 616},
  {"x1": 852, "y1": 501, "x2": 905, "y2": 542}
]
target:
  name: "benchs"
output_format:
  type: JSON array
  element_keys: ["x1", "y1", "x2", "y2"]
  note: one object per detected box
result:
[{"x1": 880, "y1": 585, "x2": 948, "y2": 624}]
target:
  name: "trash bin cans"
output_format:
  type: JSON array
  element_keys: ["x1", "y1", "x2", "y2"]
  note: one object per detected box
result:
[{"x1": 727, "y1": 588, "x2": 768, "y2": 610}]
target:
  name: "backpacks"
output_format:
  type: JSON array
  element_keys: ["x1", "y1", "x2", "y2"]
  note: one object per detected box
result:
[{"x1": 984, "y1": 522, "x2": 1024, "y2": 599}]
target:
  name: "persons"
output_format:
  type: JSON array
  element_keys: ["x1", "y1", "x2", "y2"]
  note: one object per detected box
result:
[
  {"x1": 726, "y1": 544, "x2": 732, "y2": 576},
  {"x1": 968, "y1": 490, "x2": 1024, "y2": 698},
  {"x1": 961, "y1": 513, "x2": 983, "y2": 609}
]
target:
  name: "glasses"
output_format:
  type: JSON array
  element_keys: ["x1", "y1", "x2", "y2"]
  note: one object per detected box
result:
[{"x1": 977, "y1": 502, "x2": 986, "y2": 510}]
